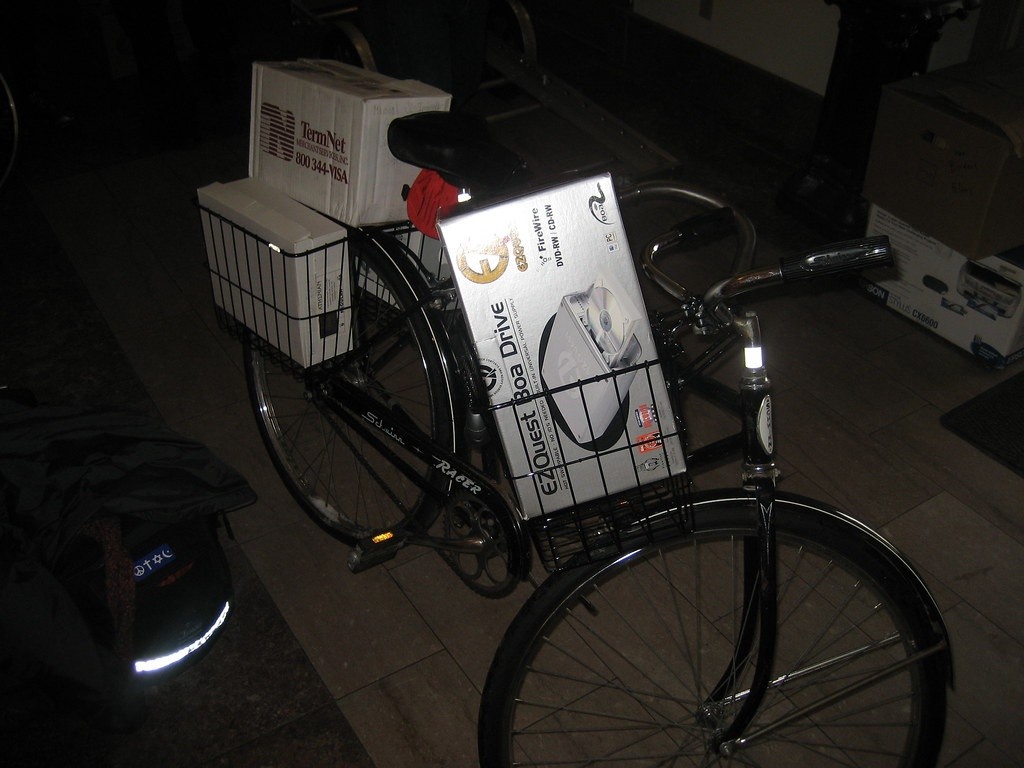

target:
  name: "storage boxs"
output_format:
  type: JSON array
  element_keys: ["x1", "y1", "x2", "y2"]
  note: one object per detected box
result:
[
  {"x1": 247, "y1": 59, "x2": 452, "y2": 228},
  {"x1": 436, "y1": 170, "x2": 687, "y2": 522},
  {"x1": 197, "y1": 178, "x2": 353, "y2": 369},
  {"x1": 356, "y1": 223, "x2": 460, "y2": 312},
  {"x1": 861, "y1": 48, "x2": 1024, "y2": 261},
  {"x1": 854, "y1": 202, "x2": 1024, "y2": 371}
]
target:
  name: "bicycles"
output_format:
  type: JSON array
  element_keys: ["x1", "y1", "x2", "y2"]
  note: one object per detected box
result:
[{"x1": 188, "y1": 109, "x2": 956, "y2": 768}]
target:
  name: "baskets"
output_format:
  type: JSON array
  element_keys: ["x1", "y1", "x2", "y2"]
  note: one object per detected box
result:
[
  {"x1": 487, "y1": 355, "x2": 697, "y2": 574},
  {"x1": 348, "y1": 218, "x2": 456, "y2": 339},
  {"x1": 194, "y1": 179, "x2": 360, "y2": 375}
]
